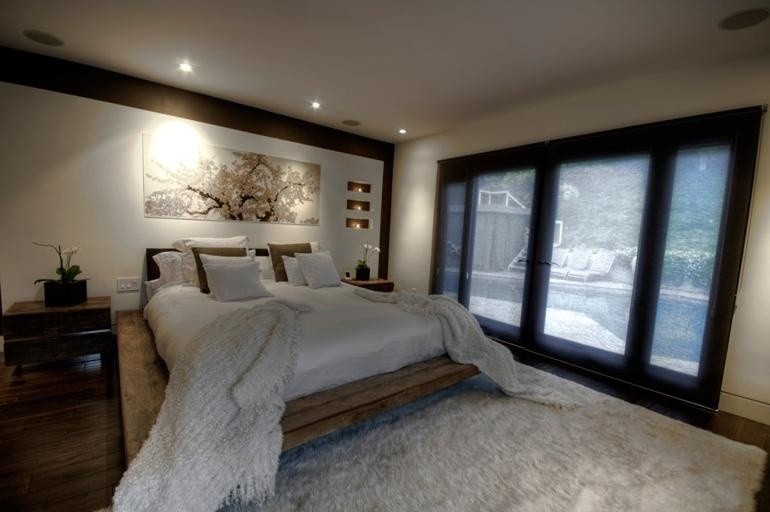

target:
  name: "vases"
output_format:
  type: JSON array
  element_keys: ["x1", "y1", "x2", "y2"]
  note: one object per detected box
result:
[
  {"x1": 43, "y1": 282, "x2": 88, "y2": 307},
  {"x1": 355, "y1": 268, "x2": 370, "y2": 281}
]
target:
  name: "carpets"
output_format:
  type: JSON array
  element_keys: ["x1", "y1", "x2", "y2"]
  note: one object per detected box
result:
[{"x1": 214, "y1": 360, "x2": 768, "y2": 512}]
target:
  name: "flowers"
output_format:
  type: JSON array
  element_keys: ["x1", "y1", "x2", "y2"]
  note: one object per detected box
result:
[
  {"x1": 31, "y1": 241, "x2": 92, "y2": 285},
  {"x1": 352, "y1": 240, "x2": 380, "y2": 271}
]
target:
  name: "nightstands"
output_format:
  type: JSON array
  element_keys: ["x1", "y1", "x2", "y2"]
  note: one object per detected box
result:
[
  {"x1": 341, "y1": 278, "x2": 394, "y2": 293},
  {"x1": 1, "y1": 296, "x2": 113, "y2": 367}
]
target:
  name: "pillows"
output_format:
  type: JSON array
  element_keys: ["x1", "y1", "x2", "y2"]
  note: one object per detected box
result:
[
  {"x1": 172, "y1": 235, "x2": 249, "y2": 288},
  {"x1": 281, "y1": 255, "x2": 307, "y2": 286},
  {"x1": 153, "y1": 251, "x2": 188, "y2": 284},
  {"x1": 191, "y1": 247, "x2": 246, "y2": 295},
  {"x1": 266, "y1": 242, "x2": 320, "y2": 283},
  {"x1": 294, "y1": 251, "x2": 342, "y2": 288},
  {"x1": 199, "y1": 252, "x2": 255, "y2": 298},
  {"x1": 205, "y1": 261, "x2": 274, "y2": 301}
]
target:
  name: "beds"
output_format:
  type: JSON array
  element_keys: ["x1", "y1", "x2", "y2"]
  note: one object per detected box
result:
[{"x1": 141, "y1": 240, "x2": 500, "y2": 465}]
